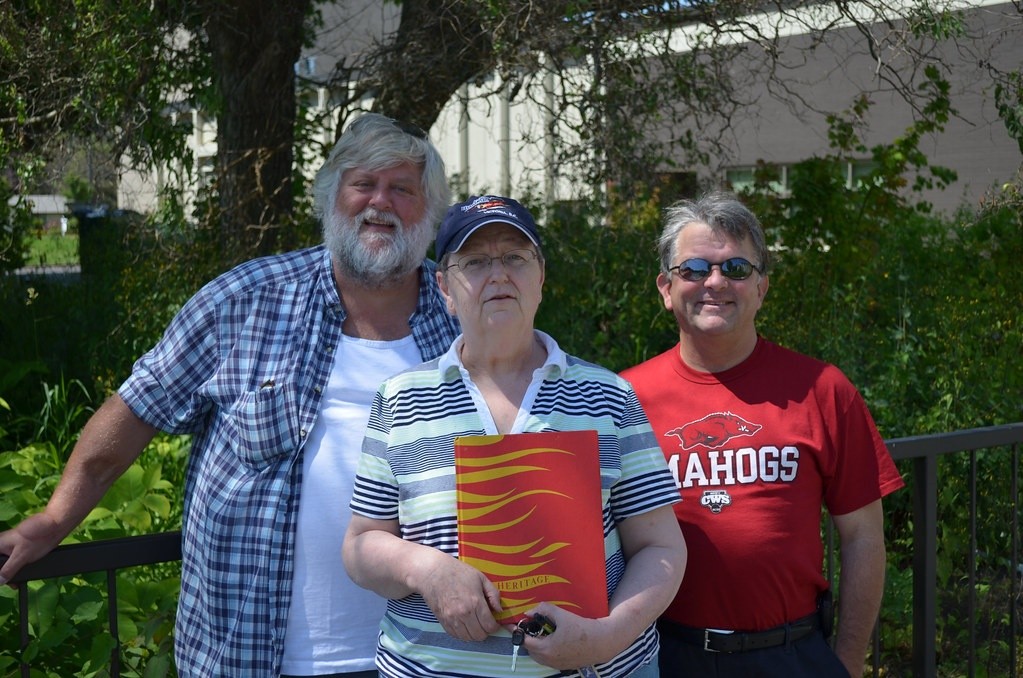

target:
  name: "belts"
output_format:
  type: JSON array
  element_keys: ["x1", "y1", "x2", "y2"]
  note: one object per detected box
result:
[{"x1": 656, "y1": 617, "x2": 816, "y2": 652}]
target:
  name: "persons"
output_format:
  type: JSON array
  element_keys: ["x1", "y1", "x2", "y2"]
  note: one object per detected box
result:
[
  {"x1": 343, "y1": 194, "x2": 688, "y2": 678},
  {"x1": 0, "y1": 116, "x2": 463, "y2": 677},
  {"x1": 619, "y1": 196, "x2": 904, "y2": 678}
]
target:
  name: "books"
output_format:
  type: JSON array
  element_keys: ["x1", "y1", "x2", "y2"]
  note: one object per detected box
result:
[{"x1": 455, "y1": 431, "x2": 609, "y2": 636}]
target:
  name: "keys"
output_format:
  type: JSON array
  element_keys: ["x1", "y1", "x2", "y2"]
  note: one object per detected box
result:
[
  {"x1": 512, "y1": 630, "x2": 524, "y2": 672},
  {"x1": 525, "y1": 621, "x2": 542, "y2": 637}
]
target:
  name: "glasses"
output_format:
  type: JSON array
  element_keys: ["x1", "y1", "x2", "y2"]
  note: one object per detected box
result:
[
  {"x1": 444, "y1": 249, "x2": 538, "y2": 274},
  {"x1": 667, "y1": 257, "x2": 762, "y2": 280}
]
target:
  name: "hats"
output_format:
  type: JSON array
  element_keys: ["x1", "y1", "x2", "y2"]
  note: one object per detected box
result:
[{"x1": 435, "y1": 195, "x2": 541, "y2": 263}]
